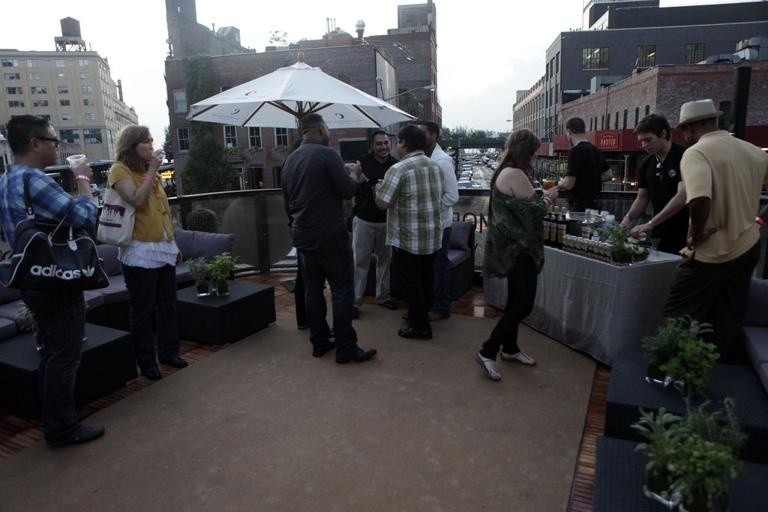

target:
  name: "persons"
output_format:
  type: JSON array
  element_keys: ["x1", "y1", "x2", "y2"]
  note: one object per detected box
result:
[
  {"x1": 352, "y1": 128, "x2": 398, "y2": 320},
  {"x1": 618, "y1": 113, "x2": 690, "y2": 253},
  {"x1": 106, "y1": 125, "x2": 188, "y2": 381},
  {"x1": 0, "y1": 115, "x2": 106, "y2": 449},
  {"x1": 279, "y1": 114, "x2": 377, "y2": 365},
  {"x1": 475, "y1": 128, "x2": 559, "y2": 381},
  {"x1": 558, "y1": 117, "x2": 609, "y2": 212},
  {"x1": 371, "y1": 125, "x2": 446, "y2": 339},
  {"x1": 654, "y1": 99, "x2": 767, "y2": 359}
]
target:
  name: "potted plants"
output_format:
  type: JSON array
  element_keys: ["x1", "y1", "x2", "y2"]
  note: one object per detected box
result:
[
  {"x1": 206, "y1": 248, "x2": 241, "y2": 299},
  {"x1": 14, "y1": 297, "x2": 44, "y2": 357},
  {"x1": 627, "y1": 389, "x2": 757, "y2": 509},
  {"x1": 183, "y1": 254, "x2": 215, "y2": 299},
  {"x1": 632, "y1": 308, "x2": 725, "y2": 407}
]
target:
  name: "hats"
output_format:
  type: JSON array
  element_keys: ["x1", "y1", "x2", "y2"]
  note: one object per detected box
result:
[{"x1": 673, "y1": 99, "x2": 724, "y2": 130}]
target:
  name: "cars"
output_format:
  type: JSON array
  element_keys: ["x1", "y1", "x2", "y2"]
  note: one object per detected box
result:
[
  {"x1": 89, "y1": 183, "x2": 101, "y2": 195},
  {"x1": 456, "y1": 148, "x2": 500, "y2": 204}
]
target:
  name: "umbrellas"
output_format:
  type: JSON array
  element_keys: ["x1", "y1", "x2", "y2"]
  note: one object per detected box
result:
[{"x1": 185, "y1": 52, "x2": 418, "y2": 138}]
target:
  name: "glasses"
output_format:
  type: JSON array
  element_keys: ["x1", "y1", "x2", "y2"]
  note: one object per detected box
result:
[
  {"x1": 655, "y1": 162, "x2": 664, "y2": 186},
  {"x1": 27, "y1": 136, "x2": 59, "y2": 148}
]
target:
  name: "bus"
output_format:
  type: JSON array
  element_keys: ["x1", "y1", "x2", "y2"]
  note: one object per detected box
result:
[
  {"x1": 48, "y1": 160, "x2": 116, "y2": 194},
  {"x1": 44, "y1": 173, "x2": 63, "y2": 189}
]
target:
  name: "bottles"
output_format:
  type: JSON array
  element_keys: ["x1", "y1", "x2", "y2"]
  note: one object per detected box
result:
[
  {"x1": 539, "y1": 204, "x2": 565, "y2": 249},
  {"x1": 630, "y1": 229, "x2": 647, "y2": 241},
  {"x1": 582, "y1": 207, "x2": 615, "y2": 240}
]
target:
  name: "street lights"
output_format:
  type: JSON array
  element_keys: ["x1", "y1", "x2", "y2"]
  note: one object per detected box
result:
[
  {"x1": 374, "y1": 75, "x2": 438, "y2": 101},
  {"x1": 506, "y1": 119, "x2": 531, "y2": 129}
]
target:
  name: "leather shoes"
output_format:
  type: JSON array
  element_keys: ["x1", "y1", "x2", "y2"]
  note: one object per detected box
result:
[
  {"x1": 312, "y1": 338, "x2": 335, "y2": 357},
  {"x1": 376, "y1": 300, "x2": 398, "y2": 310},
  {"x1": 335, "y1": 349, "x2": 376, "y2": 363},
  {"x1": 402, "y1": 313, "x2": 407, "y2": 320},
  {"x1": 399, "y1": 327, "x2": 432, "y2": 340},
  {"x1": 351, "y1": 306, "x2": 359, "y2": 319},
  {"x1": 166, "y1": 355, "x2": 187, "y2": 369}
]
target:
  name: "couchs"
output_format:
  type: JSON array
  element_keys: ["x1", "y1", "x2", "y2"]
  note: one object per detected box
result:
[
  {"x1": 366, "y1": 217, "x2": 477, "y2": 313},
  {"x1": 0, "y1": 227, "x2": 111, "y2": 345},
  {"x1": 80, "y1": 226, "x2": 238, "y2": 337}
]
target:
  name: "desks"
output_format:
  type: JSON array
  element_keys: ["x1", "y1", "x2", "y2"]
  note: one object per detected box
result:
[
  {"x1": 170, "y1": 276, "x2": 275, "y2": 352},
  {"x1": 475, "y1": 223, "x2": 684, "y2": 374},
  {"x1": 592, "y1": 432, "x2": 768, "y2": 511},
  {"x1": 600, "y1": 344, "x2": 767, "y2": 468},
  {"x1": 0, "y1": 316, "x2": 139, "y2": 428}
]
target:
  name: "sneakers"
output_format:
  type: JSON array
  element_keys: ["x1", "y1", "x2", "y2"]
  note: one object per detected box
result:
[
  {"x1": 46, "y1": 425, "x2": 105, "y2": 448},
  {"x1": 142, "y1": 365, "x2": 161, "y2": 380},
  {"x1": 475, "y1": 351, "x2": 501, "y2": 380},
  {"x1": 500, "y1": 350, "x2": 536, "y2": 366},
  {"x1": 427, "y1": 311, "x2": 447, "y2": 321}
]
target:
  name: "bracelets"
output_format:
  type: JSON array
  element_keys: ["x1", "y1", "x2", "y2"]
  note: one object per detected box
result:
[
  {"x1": 625, "y1": 214, "x2": 633, "y2": 220},
  {"x1": 73, "y1": 175, "x2": 91, "y2": 182},
  {"x1": 755, "y1": 216, "x2": 765, "y2": 225},
  {"x1": 143, "y1": 174, "x2": 155, "y2": 185},
  {"x1": 648, "y1": 220, "x2": 656, "y2": 225}
]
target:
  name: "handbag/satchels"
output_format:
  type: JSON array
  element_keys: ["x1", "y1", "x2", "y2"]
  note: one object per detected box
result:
[
  {"x1": 96, "y1": 175, "x2": 137, "y2": 248},
  {"x1": 1, "y1": 171, "x2": 111, "y2": 291}
]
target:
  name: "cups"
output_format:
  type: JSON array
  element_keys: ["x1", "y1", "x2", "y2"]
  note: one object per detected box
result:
[
  {"x1": 68, "y1": 153, "x2": 89, "y2": 186},
  {"x1": 375, "y1": 178, "x2": 386, "y2": 197},
  {"x1": 151, "y1": 144, "x2": 168, "y2": 162},
  {"x1": 344, "y1": 161, "x2": 361, "y2": 188},
  {"x1": 540, "y1": 178, "x2": 559, "y2": 202}
]
